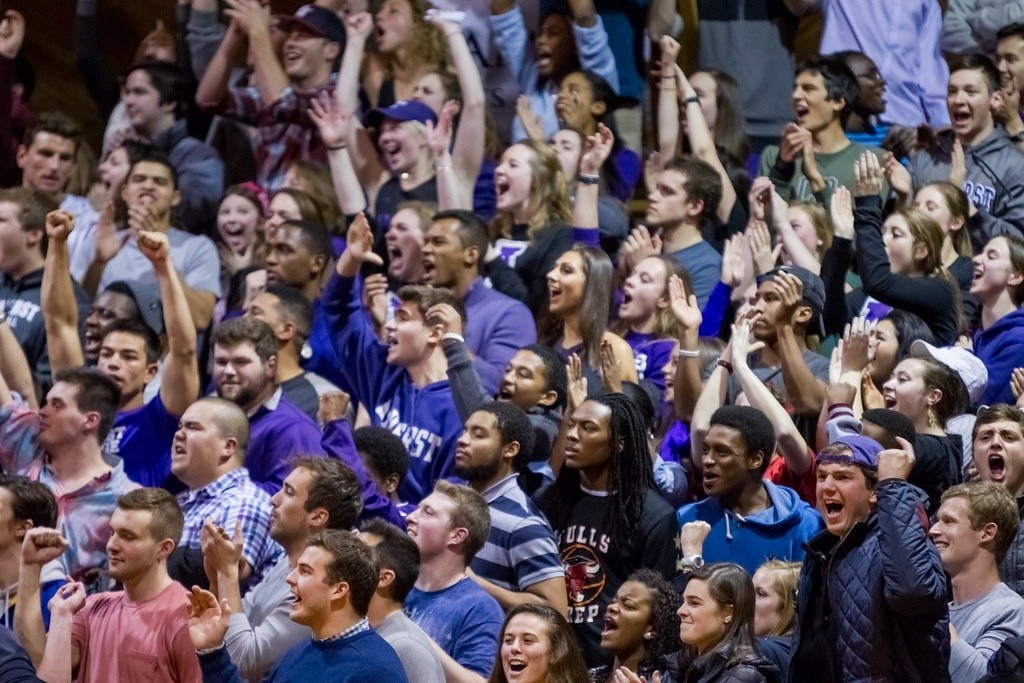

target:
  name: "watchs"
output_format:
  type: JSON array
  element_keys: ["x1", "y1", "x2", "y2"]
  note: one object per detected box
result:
[
  {"x1": 712, "y1": 358, "x2": 733, "y2": 375},
  {"x1": 679, "y1": 554, "x2": 704, "y2": 571}
]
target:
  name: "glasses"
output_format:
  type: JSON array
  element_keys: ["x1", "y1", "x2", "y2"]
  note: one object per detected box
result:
[{"x1": 858, "y1": 70, "x2": 880, "y2": 81}]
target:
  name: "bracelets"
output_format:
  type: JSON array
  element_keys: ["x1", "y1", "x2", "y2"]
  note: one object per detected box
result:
[
  {"x1": 1008, "y1": 133, "x2": 1023, "y2": 143},
  {"x1": 579, "y1": 173, "x2": 600, "y2": 185},
  {"x1": 440, "y1": 30, "x2": 464, "y2": 46},
  {"x1": 650, "y1": 69, "x2": 678, "y2": 92},
  {"x1": 441, "y1": 332, "x2": 465, "y2": 346},
  {"x1": 676, "y1": 349, "x2": 700, "y2": 357},
  {"x1": 683, "y1": 97, "x2": 698, "y2": 104},
  {"x1": 435, "y1": 166, "x2": 452, "y2": 171},
  {"x1": 326, "y1": 145, "x2": 344, "y2": 153}
]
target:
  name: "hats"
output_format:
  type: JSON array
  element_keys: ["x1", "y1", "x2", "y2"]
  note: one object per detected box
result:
[
  {"x1": 911, "y1": 338, "x2": 988, "y2": 407},
  {"x1": 105, "y1": 280, "x2": 166, "y2": 336},
  {"x1": 273, "y1": 4, "x2": 345, "y2": 48},
  {"x1": 755, "y1": 264, "x2": 828, "y2": 336},
  {"x1": 361, "y1": 100, "x2": 438, "y2": 128},
  {"x1": 815, "y1": 435, "x2": 888, "y2": 470}
]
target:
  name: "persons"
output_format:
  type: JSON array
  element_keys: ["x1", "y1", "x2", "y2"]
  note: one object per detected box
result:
[{"x1": 1, "y1": 1, "x2": 1024, "y2": 681}]
